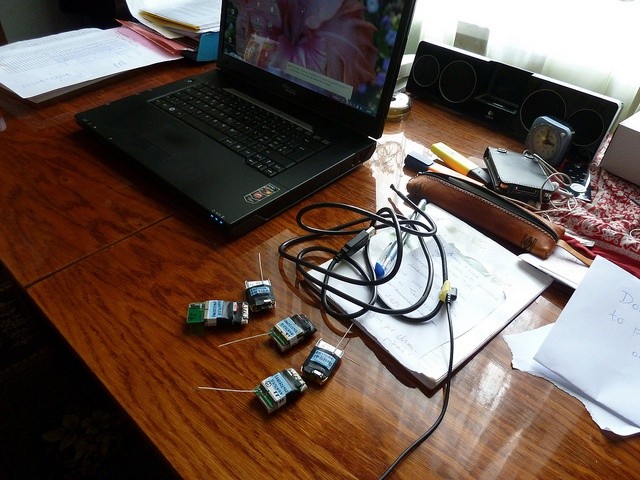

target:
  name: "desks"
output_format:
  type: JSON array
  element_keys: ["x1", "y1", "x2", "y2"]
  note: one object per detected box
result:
[{"x1": 0, "y1": 56, "x2": 639, "y2": 479}]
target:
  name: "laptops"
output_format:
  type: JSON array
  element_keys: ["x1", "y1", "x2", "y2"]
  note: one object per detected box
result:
[{"x1": 71, "y1": 1, "x2": 414, "y2": 231}]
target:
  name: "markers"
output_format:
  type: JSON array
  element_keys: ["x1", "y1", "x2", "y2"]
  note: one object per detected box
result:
[
  {"x1": 404, "y1": 149, "x2": 489, "y2": 193},
  {"x1": 430, "y1": 142, "x2": 494, "y2": 189}
]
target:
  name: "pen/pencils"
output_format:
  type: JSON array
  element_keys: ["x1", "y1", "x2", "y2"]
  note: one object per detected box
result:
[{"x1": 375, "y1": 198, "x2": 427, "y2": 279}]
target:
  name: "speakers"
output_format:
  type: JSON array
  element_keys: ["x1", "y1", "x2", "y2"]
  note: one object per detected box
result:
[{"x1": 405, "y1": 40, "x2": 621, "y2": 165}]
